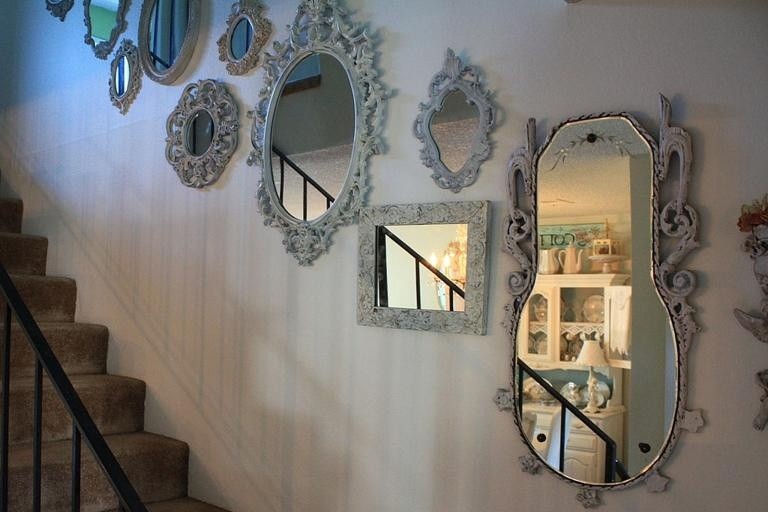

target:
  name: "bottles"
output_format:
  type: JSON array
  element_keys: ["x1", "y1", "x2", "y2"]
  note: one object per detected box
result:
[{"x1": 528, "y1": 333, "x2": 604, "y2": 361}]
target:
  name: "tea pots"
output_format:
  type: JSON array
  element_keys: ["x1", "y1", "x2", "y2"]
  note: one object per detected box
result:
[
  {"x1": 537, "y1": 247, "x2": 560, "y2": 274},
  {"x1": 558, "y1": 241, "x2": 584, "y2": 273}
]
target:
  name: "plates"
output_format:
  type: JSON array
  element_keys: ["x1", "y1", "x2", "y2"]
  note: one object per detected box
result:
[
  {"x1": 590, "y1": 255, "x2": 625, "y2": 274},
  {"x1": 535, "y1": 294, "x2": 566, "y2": 323},
  {"x1": 522, "y1": 376, "x2": 610, "y2": 408},
  {"x1": 581, "y1": 295, "x2": 606, "y2": 323}
]
